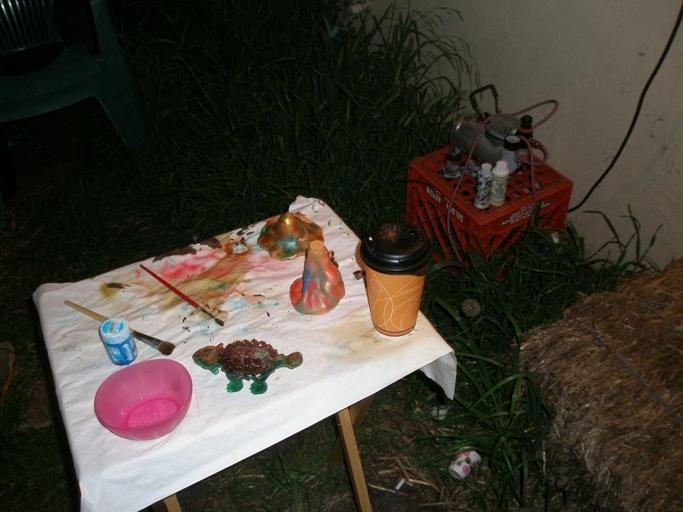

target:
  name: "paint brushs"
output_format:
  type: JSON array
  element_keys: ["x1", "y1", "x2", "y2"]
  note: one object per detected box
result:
[
  {"x1": 64, "y1": 300, "x2": 175, "y2": 355},
  {"x1": 140, "y1": 264, "x2": 224, "y2": 326}
]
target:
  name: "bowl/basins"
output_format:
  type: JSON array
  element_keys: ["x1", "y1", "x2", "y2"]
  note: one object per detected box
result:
[{"x1": 92, "y1": 356, "x2": 194, "y2": 443}]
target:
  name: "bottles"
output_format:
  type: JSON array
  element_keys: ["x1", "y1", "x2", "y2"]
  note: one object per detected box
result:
[
  {"x1": 98, "y1": 317, "x2": 137, "y2": 364},
  {"x1": 517, "y1": 115, "x2": 533, "y2": 160},
  {"x1": 491, "y1": 160, "x2": 510, "y2": 209},
  {"x1": 501, "y1": 135, "x2": 521, "y2": 174},
  {"x1": 473, "y1": 162, "x2": 491, "y2": 210}
]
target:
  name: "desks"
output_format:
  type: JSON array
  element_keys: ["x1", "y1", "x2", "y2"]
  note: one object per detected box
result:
[{"x1": 34, "y1": 195, "x2": 452, "y2": 512}]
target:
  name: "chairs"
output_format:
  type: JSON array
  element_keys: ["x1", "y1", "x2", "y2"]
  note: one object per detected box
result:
[{"x1": 1, "y1": 0, "x2": 146, "y2": 150}]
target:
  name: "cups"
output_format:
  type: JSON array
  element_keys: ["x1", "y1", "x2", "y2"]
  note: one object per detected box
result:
[{"x1": 357, "y1": 220, "x2": 429, "y2": 337}]
